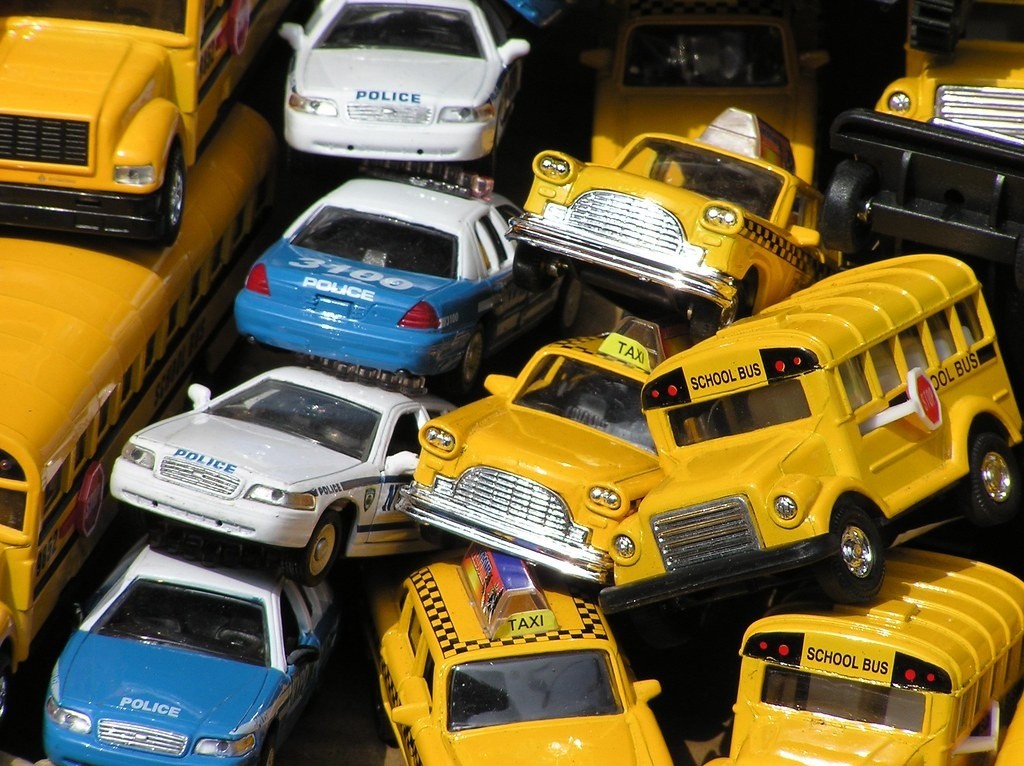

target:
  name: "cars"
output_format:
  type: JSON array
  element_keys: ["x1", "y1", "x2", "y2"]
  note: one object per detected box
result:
[
  {"x1": 576, "y1": 1, "x2": 827, "y2": 232},
  {"x1": 231, "y1": 162, "x2": 577, "y2": 402},
  {"x1": 815, "y1": 1, "x2": 1024, "y2": 305},
  {"x1": 358, "y1": 552, "x2": 679, "y2": 766},
  {"x1": 395, "y1": 315, "x2": 684, "y2": 586},
  {"x1": 502, "y1": 107, "x2": 840, "y2": 348},
  {"x1": 105, "y1": 363, "x2": 454, "y2": 587},
  {"x1": 274, "y1": 2, "x2": 532, "y2": 165},
  {"x1": 39, "y1": 530, "x2": 350, "y2": 766}
]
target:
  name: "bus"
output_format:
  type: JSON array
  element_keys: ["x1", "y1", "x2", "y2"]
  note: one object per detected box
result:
[
  {"x1": 590, "y1": 251, "x2": 1024, "y2": 650},
  {"x1": 1, "y1": 0, "x2": 292, "y2": 250},
  {"x1": 681, "y1": 546, "x2": 1024, "y2": 766},
  {"x1": 1, "y1": 99, "x2": 285, "y2": 735}
]
